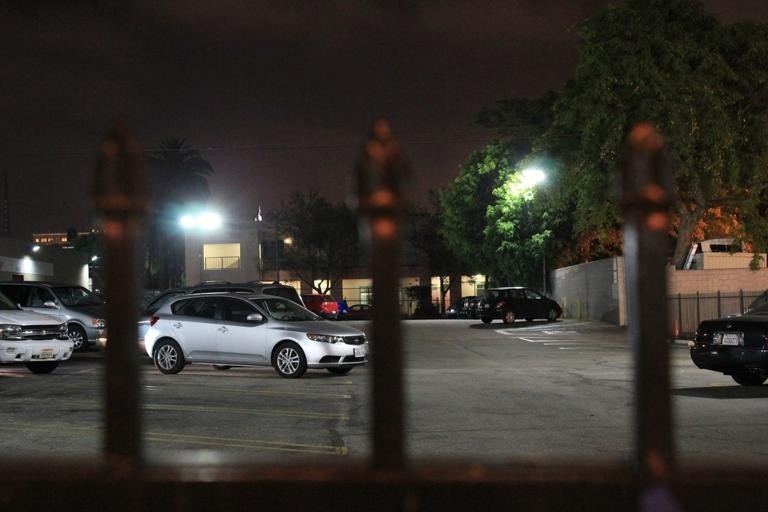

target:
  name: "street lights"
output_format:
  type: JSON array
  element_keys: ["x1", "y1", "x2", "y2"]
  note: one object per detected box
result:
[{"x1": 503, "y1": 162, "x2": 548, "y2": 294}]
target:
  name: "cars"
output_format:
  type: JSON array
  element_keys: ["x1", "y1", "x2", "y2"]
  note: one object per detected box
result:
[
  {"x1": 443, "y1": 282, "x2": 567, "y2": 331},
  {"x1": 689, "y1": 286, "x2": 768, "y2": 387}
]
